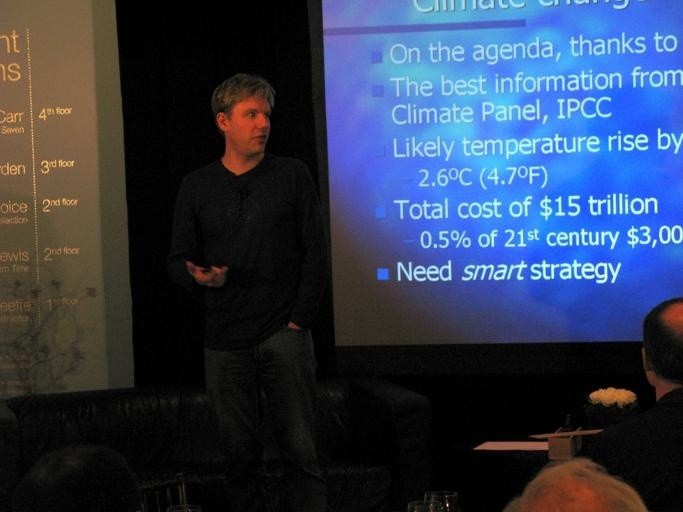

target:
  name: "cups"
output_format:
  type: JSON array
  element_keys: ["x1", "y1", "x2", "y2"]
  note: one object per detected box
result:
[{"x1": 407, "y1": 489, "x2": 458, "y2": 512}]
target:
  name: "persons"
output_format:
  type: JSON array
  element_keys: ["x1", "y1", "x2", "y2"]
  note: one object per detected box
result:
[
  {"x1": 575, "y1": 296, "x2": 683, "y2": 512},
  {"x1": 15, "y1": 434, "x2": 144, "y2": 512},
  {"x1": 501, "y1": 455, "x2": 648, "y2": 512},
  {"x1": 166, "y1": 72, "x2": 329, "y2": 511}
]
